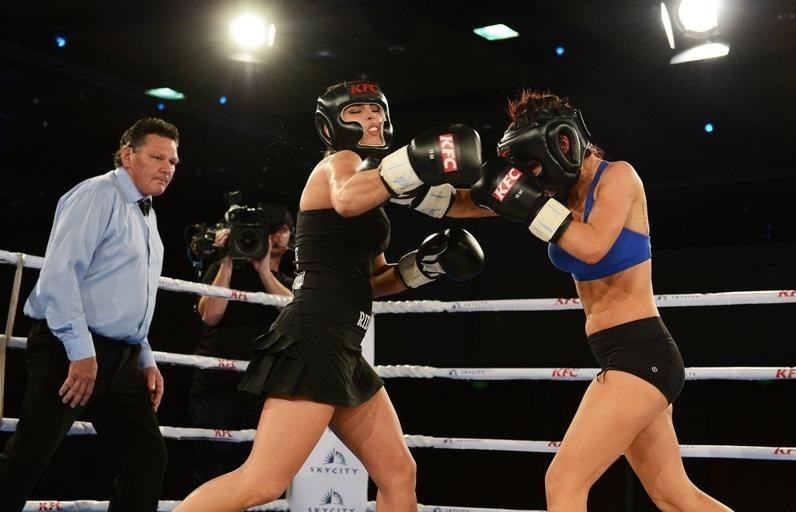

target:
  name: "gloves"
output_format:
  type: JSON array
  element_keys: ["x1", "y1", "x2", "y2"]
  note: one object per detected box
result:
[
  {"x1": 356, "y1": 122, "x2": 483, "y2": 221},
  {"x1": 397, "y1": 224, "x2": 485, "y2": 290},
  {"x1": 469, "y1": 154, "x2": 574, "y2": 244}
]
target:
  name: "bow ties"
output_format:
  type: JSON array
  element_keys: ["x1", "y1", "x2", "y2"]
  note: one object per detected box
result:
[{"x1": 136, "y1": 197, "x2": 151, "y2": 218}]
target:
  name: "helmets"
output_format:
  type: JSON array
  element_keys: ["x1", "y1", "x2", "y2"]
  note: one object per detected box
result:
[
  {"x1": 314, "y1": 80, "x2": 396, "y2": 158},
  {"x1": 493, "y1": 105, "x2": 594, "y2": 198}
]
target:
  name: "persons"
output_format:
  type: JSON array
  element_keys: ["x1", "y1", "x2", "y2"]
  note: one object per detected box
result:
[
  {"x1": 0, "y1": 117, "x2": 181, "y2": 512},
  {"x1": 358, "y1": 90, "x2": 737, "y2": 512},
  {"x1": 169, "y1": 79, "x2": 488, "y2": 511},
  {"x1": 169, "y1": 202, "x2": 295, "y2": 500}
]
